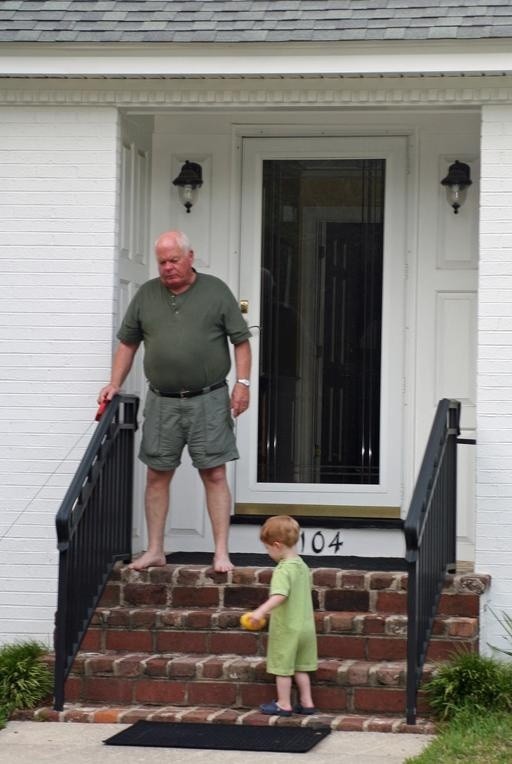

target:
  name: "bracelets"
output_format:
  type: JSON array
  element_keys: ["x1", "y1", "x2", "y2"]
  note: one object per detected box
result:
[{"x1": 236, "y1": 377, "x2": 253, "y2": 387}]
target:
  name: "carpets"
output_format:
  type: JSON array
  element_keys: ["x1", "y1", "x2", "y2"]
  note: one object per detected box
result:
[
  {"x1": 167, "y1": 548, "x2": 415, "y2": 573},
  {"x1": 100, "y1": 720, "x2": 336, "y2": 753}
]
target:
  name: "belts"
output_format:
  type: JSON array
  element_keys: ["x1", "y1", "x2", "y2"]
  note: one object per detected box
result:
[{"x1": 148, "y1": 380, "x2": 228, "y2": 398}]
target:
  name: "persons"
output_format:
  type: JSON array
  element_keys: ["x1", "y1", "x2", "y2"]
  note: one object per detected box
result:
[
  {"x1": 97, "y1": 231, "x2": 255, "y2": 574},
  {"x1": 244, "y1": 513, "x2": 321, "y2": 718}
]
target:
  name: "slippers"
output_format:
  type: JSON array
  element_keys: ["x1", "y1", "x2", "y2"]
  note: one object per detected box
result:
[
  {"x1": 294, "y1": 703, "x2": 317, "y2": 714},
  {"x1": 258, "y1": 698, "x2": 293, "y2": 717}
]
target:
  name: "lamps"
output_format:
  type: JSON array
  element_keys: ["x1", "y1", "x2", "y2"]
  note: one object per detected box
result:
[
  {"x1": 173, "y1": 159, "x2": 203, "y2": 213},
  {"x1": 440, "y1": 158, "x2": 472, "y2": 215}
]
